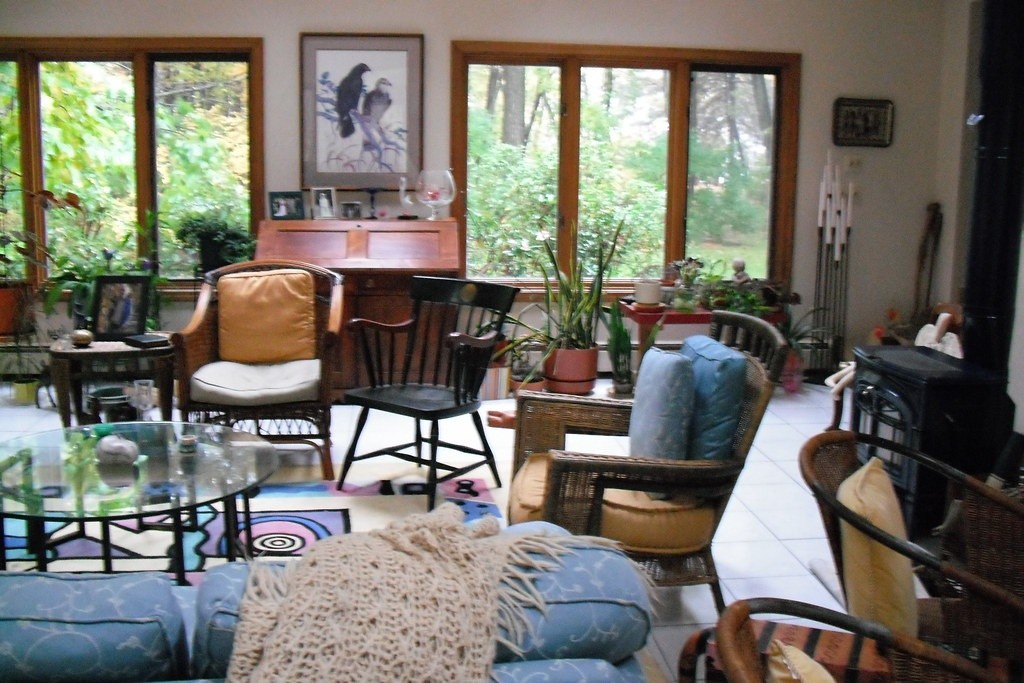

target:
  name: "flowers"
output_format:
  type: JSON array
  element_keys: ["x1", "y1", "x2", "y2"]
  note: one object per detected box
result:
[
  {"x1": 669, "y1": 257, "x2": 707, "y2": 289},
  {"x1": 0, "y1": 188, "x2": 182, "y2": 332},
  {"x1": 630, "y1": 257, "x2": 661, "y2": 283}
]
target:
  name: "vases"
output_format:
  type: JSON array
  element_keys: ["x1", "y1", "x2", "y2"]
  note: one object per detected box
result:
[
  {"x1": 674, "y1": 285, "x2": 694, "y2": 312},
  {"x1": 71, "y1": 303, "x2": 93, "y2": 331},
  {"x1": 0, "y1": 282, "x2": 28, "y2": 333},
  {"x1": 634, "y1": 282, "x2": 660, "y2": 304}
]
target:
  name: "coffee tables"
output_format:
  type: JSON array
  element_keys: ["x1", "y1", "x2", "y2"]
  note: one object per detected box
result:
[{"x1": 0, "y1": 422, "x2": 279, "y2": 587}]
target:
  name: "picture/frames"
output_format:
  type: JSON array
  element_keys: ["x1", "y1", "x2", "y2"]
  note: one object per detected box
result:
[
  {"x1": 87, "y1": 275, "x2": 151, "y2": 339},
  {"x1": 832, "y1": 97, "x2": 894, "y2": 148},
  {"x1": 309, "y1": 187, "x2": 339, "y2": 220},
  {"x1": 300, "y1": 32, "x2": 424, "y2": 192},
  {"x1": 268, "y1": 191, "x2": 305, "y2": 220}
]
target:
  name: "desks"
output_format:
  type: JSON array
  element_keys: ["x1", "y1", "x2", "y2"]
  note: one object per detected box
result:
[
  {"x1": 621, "y1": 301, "x2": 789, "y2": 366},
  {"x1": 583, "y1": 385, "x2": 635, "y2": 401},
  {"x1": 50, "y1": 331, "x2": 177, "y2": 442}
]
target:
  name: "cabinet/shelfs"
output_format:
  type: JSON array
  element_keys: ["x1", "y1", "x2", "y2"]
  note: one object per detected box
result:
[
  {"x1": 257, "y1": 218, "x2": 461, "y2": 404},
  {"x1": 850, "y1": 345, "x2": 1016, "y2": 542}
]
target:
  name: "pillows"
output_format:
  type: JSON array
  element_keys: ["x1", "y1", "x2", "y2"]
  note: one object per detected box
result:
[
  {"x1": 218, "y1": 268, "x2": 316, "y2": 362},
  {"x1": 681, "y1": 335, "x2": 747, "y2": 461},
  {"x1": 837, "y1": 457, "x2": 917, "y2": 641},
  {"x1": 629, "y1": 346, "x2": 694, "y2": 500},
  {"x1": 765, "y1": 638, "x2": 836, "y2": 682}
]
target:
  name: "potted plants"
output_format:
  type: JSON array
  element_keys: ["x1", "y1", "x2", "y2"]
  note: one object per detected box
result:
[
  {"x1": 175, "y1": 205, "x2": 258, "y2": 271},
  {"x1": 0, "y1": 278, "x2": 69, "y2": 404},
  {"x1": 608, "y1": 300, "x2": 666, "y2": 393},
  {"x1": 473, "y1": 217, "x2": 626, "y2": 394},
  {"x1": 695, "y1": 256, "x2": 835, "y2": 362}
]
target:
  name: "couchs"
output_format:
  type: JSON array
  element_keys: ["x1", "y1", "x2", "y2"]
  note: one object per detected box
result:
[{"x1": 0, "y1": 501, "x2": 663, "y2": 683}]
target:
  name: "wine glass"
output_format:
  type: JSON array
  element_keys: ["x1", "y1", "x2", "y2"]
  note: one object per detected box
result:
[{"x1": 414, "y1": 167, "x2": 456, "y2": 222}]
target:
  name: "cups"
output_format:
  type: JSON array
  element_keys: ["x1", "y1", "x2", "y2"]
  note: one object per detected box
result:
[{"x1": 634, "y1": 281, "x2": 661, "y2": 305}]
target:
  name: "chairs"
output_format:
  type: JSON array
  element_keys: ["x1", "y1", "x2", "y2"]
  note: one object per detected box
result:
[
  {"x1": 826, "y1": 303, "x2": 965, "y2": 433},
  {"x1": 169, "y1": 259, "x2": 345, "y2": 481},
  {"x1": 509, "y1": 310, "x2": 788, "y2": 617},
  {"x1": 336, "y1": 275, "x2": 521, "y2": 514},
  {"x1": 678, "y1": 430, "x2": 1024, "y2": 682}
]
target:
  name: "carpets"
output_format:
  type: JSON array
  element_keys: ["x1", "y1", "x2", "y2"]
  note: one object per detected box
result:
[{"x1": 0, "y1": 462, "x2": 503, "y2": 585}]
